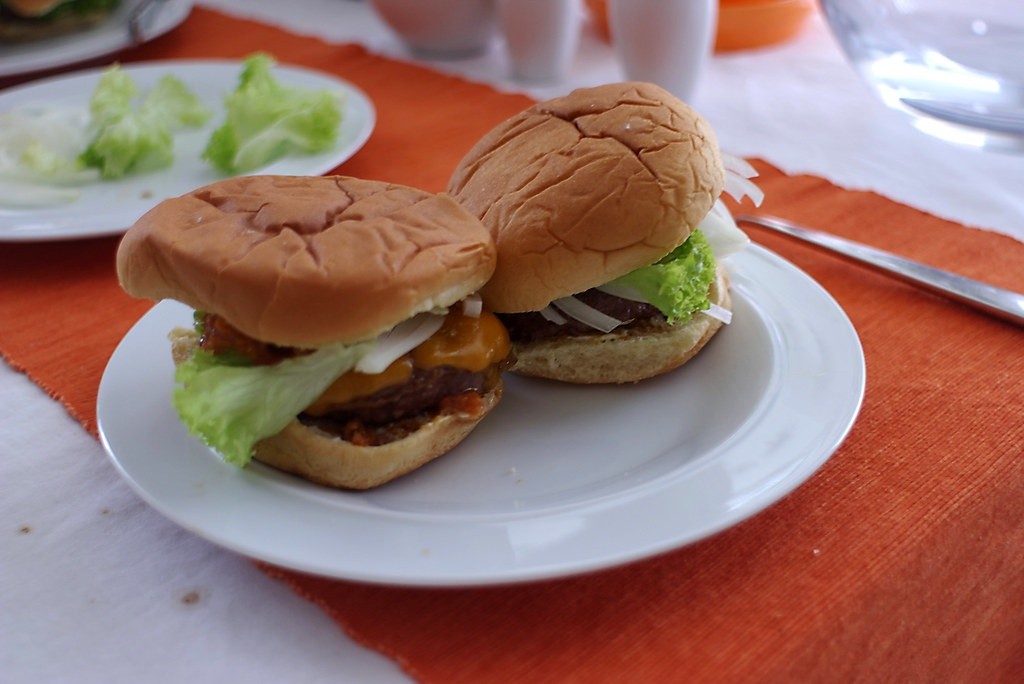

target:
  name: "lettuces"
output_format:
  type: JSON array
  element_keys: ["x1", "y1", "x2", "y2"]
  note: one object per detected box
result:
[{"x1": 10, "y1": 53, "x2": 345, "y2": 181}]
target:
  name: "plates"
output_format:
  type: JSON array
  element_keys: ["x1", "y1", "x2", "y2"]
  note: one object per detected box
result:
[
  {"x1": 95, "y1": 240, "x2": 867, "y2": 588},
  {"x1": 0, "y1": 56, "x2": 378, "y2": 241},
  {"x1": 0, "y1": 0, "x2": 196, "y2": 76}
]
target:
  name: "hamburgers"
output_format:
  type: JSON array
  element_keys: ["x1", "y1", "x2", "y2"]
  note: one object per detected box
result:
[
  {"x1": 444, "y1": 81, "x2": 766, "y2": 385},
  {"x1": 115, "y1": 174, "x2": 510, "y2": 489}
]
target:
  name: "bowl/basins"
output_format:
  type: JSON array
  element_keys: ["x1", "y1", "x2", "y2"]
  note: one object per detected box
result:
[{"x1": 375, "y1": 0, "x2": 500, "y2": 58}]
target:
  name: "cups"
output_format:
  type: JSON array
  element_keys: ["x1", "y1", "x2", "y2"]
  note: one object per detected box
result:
[
  {"x1": 499, "y1": 0, "x2": 586, "y2": 83},
  {"x1": 605, "y1": 0, "x2": 720, "y2": 112}
]
